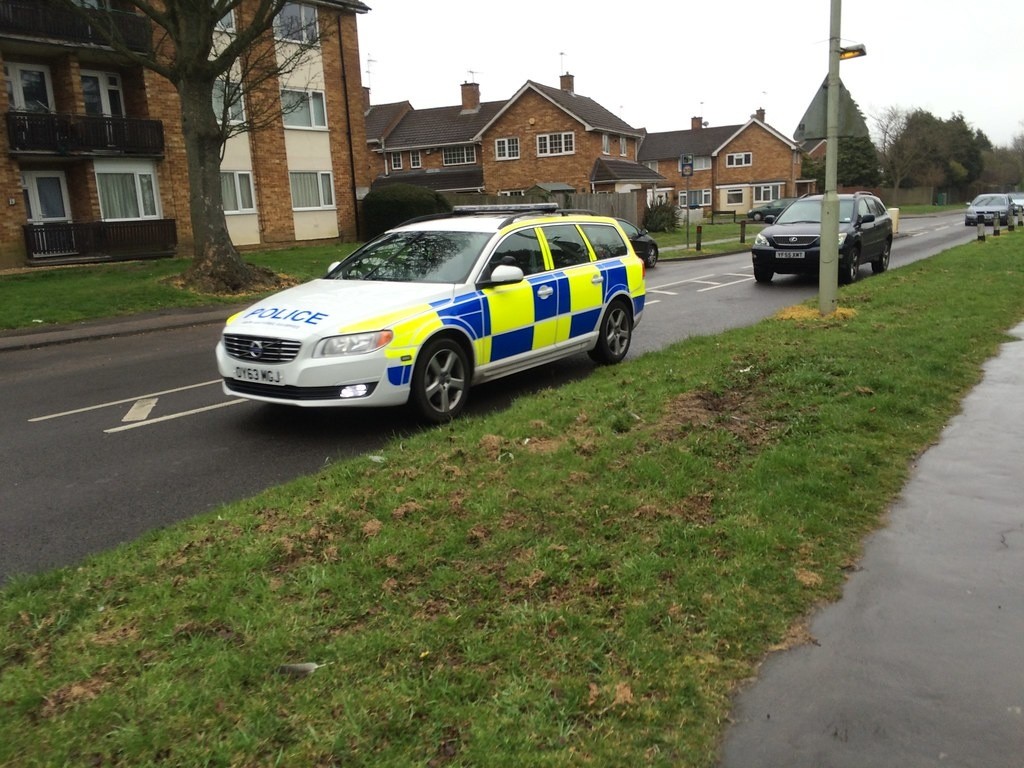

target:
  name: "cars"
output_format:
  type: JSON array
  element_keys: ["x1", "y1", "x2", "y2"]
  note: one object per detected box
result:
[
  {"x1": 611, "y1": 217, "x2": 659, "y2": 267},
  {"x1": 1005, "y1": 192, "x2": 1024, "y2": 212},
  {"x1": 747, "y1": 197, "x2": 806, "y2": 222},
  {"x1": 965, "y1": 194, "x2": 1016, "y2": 226},
  {"x1": 217, "y1": 204, "x2": 648, "y2": 425}
]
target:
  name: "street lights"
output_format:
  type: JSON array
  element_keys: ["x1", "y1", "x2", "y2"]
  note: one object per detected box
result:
[{"x1": 818, "y1": 42, "x2": 869, "y2": 322}]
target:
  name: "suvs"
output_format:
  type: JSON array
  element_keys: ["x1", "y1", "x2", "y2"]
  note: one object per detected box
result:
[{"x1": 751, "y1": 190, "x2": 895, "y2": 284}]
